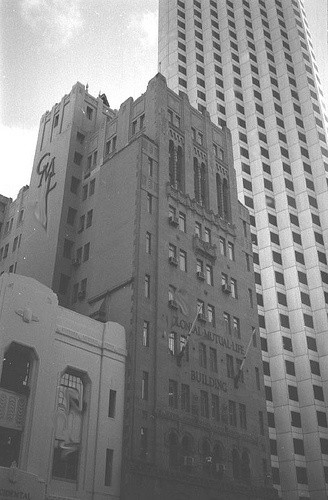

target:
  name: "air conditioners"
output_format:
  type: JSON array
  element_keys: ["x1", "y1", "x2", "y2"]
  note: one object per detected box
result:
[
  {"x1": 184, "y1": 456, "x2": 194, "y2": 467},
  {"x1": 170, "y1": 257, "x2": 178, "y2": 266},
  {"x1": 169, "y1": 216, "x2": 180, "y2": 225},
  {"x1": 197, "y1": 271, "x2": 205, "y2": 280},
  {"x1": 168, "y1": 300, "x2": 177, "y2": 307},
  {"x1": 198, "y1": 313, "x2": 205, "y2": 319},
  {"x1": 216, "y1": 463, "x2": 226, "y2": 473},
  {"x1": 223, "y1": 284, "x2": 232, "y2": 293}
]
